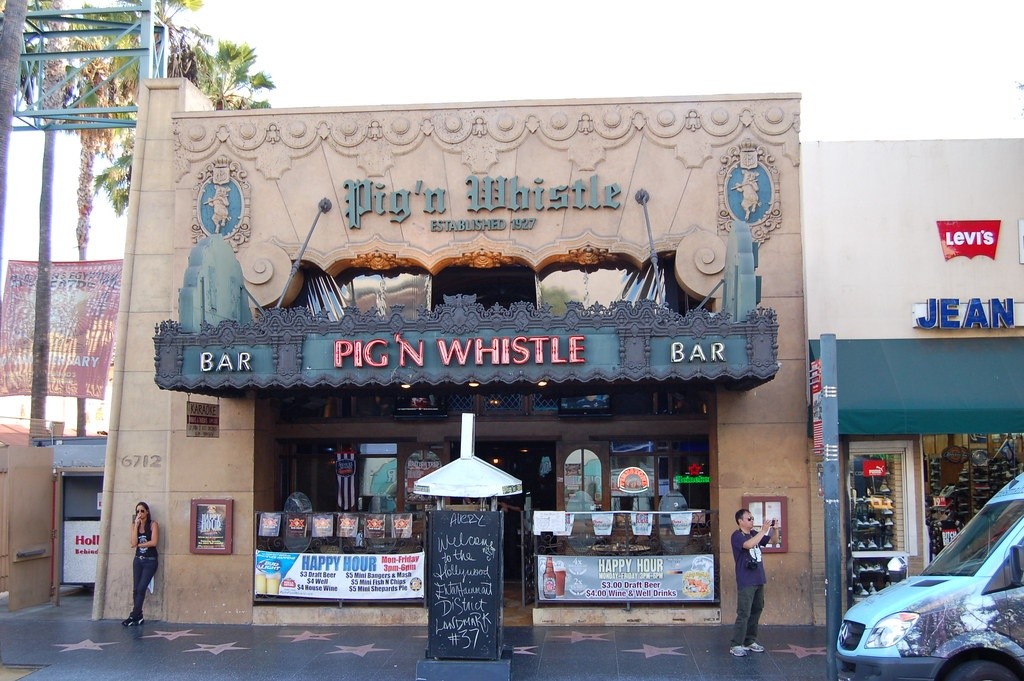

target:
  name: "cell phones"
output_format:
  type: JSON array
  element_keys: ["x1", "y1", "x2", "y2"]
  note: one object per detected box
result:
[{"x1": 770, "y1": 520, "x2": 775, "y2": 526}]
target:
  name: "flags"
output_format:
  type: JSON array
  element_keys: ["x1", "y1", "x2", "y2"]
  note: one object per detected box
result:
[{"x1": 0, "y1": 259, "x2": 124, "y2": 400}]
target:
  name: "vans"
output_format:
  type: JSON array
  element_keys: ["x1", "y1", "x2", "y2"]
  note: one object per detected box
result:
[{"x1": 836, "y1": 472, "x2": 1024, "y2": 681}]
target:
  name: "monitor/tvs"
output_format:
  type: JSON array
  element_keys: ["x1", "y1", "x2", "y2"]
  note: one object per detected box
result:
[
  {"x1": 559, "y1": 380, "x2": 613, "y2": 418},
  {"x1": 394, "y1": 383, "x2": 448, "y2": 419}
]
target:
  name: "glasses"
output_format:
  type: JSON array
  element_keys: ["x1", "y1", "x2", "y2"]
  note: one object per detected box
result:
[
  {"x1": 136, "y1": 509, "x2": 147, "y2": 513},
  {"x1": 740, "y1": 516, "x2": 754, "y2": 522}
]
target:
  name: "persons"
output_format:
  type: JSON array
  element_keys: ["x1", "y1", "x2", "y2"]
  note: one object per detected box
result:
[
  {"x1": 121, "y1": 501, "x2": 158, "y2": 626},
  {"x1": 730, "y1": 509, "x2": 778, "y2": 656}
]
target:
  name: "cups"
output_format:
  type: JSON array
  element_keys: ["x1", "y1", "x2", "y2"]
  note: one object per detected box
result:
[
  {"x1": 554, "y1": 571, "x2": 566, "y2": 596},
  {"x1": 266, "y1": 578, "x2": 278, "y2": 594},
  {"x1": 256, "y1": 575, "x2": 266, "y2": 594}
]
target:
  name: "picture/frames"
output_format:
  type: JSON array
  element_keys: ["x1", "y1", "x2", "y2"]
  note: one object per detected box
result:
[
  {"x1": 190, "y1": 498, "x2": 233, "y2": 555},
  {"x1": 742, "y1": 496, "x2": 789, "y2": 553}
]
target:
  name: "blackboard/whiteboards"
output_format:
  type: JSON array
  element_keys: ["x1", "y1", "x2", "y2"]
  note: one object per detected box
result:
[{"x1": 424, "y1": 508, "x2": 505, "y2": 660}]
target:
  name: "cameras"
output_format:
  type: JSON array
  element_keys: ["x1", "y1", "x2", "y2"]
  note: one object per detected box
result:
[{"x1": 746, "y1": 560, "x2": 758, "y2": 569}]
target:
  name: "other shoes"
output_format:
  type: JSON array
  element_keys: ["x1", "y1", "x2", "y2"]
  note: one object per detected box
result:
[
  {"x1": 857, "y1": 484, "x2": 894, "y2": 549},
  {"x1": 929, "y1": 433, "x2": 1024, "y2": 516},
  {"x1": 855, "y1": 564, "x2": 889, "y2": 596}
]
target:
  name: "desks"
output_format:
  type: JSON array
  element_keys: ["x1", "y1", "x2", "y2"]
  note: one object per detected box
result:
[{"x1": 586, "y1": 544, "x2": 650, "y2": 556}]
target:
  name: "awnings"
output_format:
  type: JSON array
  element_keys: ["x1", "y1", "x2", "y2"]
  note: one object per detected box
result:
[{"x1": 807, "y1": 336, "x2": 1024, "y2": 438}]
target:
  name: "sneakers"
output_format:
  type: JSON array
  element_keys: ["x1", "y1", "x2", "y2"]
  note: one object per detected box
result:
[
  {"x1": 742, "y1": 642, "x2": 765, "y2": 653},
  {"x1": 729, "y1": 646, "x2": 747, "y2": 657},
  {"x1": 121, "y1": 614, "x2": 144, "y2": 626}
]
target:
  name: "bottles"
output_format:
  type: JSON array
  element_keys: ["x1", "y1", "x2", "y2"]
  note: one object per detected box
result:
[{"x1": 543, "y1": 557, "x2": 556, "y2": 598}]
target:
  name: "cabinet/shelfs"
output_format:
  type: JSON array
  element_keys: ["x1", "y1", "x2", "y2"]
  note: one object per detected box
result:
[{"x1": 851, "y1": 473, "x2": 894, "y2": 602}]
